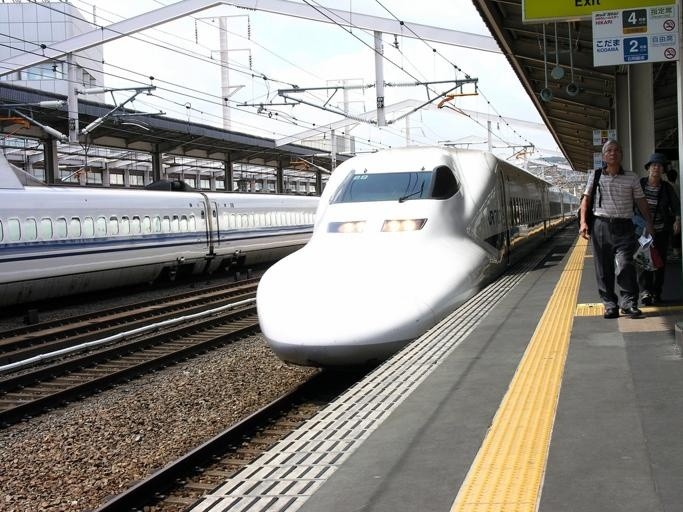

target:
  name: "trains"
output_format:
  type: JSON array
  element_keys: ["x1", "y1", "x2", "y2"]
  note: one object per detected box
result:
[
  {"x1": 253, "y1": 146, "x2": 582, "y2": 366},
  {"x1": 1, "y1": 147, "x2": 321, "y2": 304}
]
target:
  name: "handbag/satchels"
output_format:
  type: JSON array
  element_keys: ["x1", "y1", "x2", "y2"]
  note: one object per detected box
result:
[
  {"x1": 650, "y1": 241, "x2": 664, "y2": 268},
  {"x1": 633, "y1": 225, "x2": 655, "y2": 257},
  {"x1": 577, "y1": 205, "x2": 594, "y2": 235}
]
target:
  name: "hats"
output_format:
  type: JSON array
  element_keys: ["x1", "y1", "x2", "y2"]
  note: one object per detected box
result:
[{"x1": 644, "y1": 153, "x2": 668, "y2": 174}]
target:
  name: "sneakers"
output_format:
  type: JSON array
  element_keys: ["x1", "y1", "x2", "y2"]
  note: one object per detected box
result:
[
  {"x1": 640, "y1": 290, "x2": 652, "y2": 303},
  {"x1": 667, "y1": 253, "x2": 681, "y2": 260}
]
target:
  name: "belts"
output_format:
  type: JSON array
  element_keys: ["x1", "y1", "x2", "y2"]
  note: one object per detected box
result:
[{"x1": 602, "y1": 217, "x2": 627, "y2": 224}]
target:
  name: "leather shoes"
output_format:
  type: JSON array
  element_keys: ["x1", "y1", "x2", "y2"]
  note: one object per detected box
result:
[
  {"x1": 621, "y1": 307, "x2": 641, "y2": 315},
  {"x1": 604, "y1": 308, "x2": 619, "y2": 318}
]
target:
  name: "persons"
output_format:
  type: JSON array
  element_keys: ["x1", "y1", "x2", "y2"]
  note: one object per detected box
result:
[
  {"x1": 576, "y1": 137, "x2": 654, "y2": 319},
  {"x1": 667, "y1": 168, "x2": 680, "y2": 262},
  {"x1": 638, "y1": 151, "x2": 681, "y2": 307}
]
